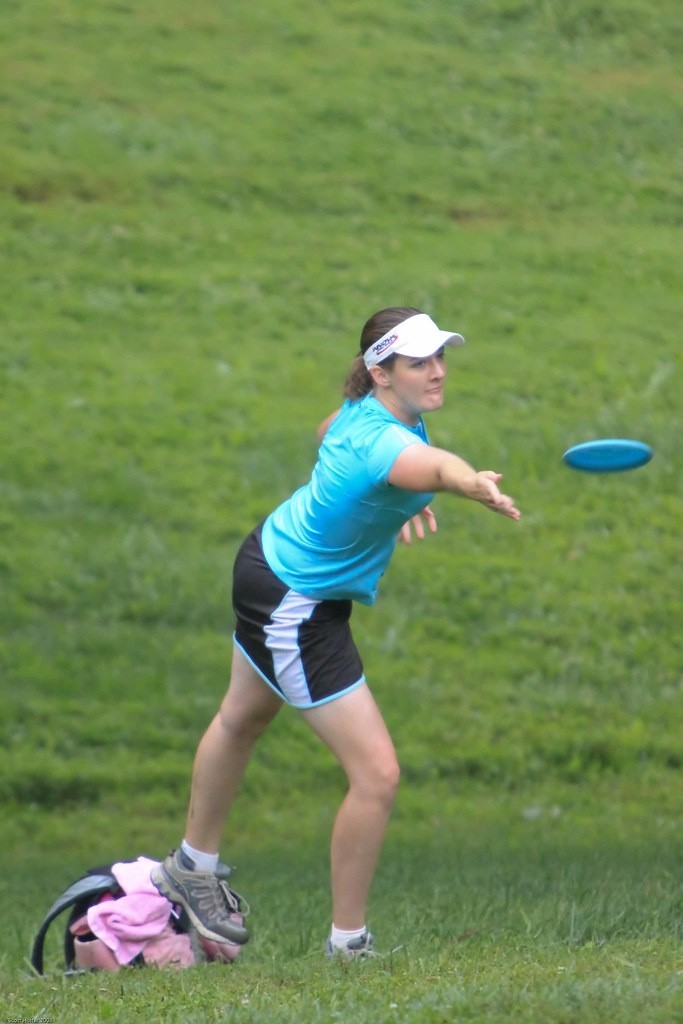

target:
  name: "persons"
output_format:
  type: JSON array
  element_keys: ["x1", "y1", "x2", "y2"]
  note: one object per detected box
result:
[{"x1": 151, "y1": 307, "x2": 520, "y2": 961}]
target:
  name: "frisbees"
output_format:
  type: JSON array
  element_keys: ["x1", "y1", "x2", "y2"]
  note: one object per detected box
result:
[{"x1": 560, "y1": 438, "x2": 654, "y2": 473}]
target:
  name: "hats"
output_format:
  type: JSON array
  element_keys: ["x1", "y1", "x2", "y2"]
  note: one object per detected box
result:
[{"x1": 363, "y1": 313, "x2": 465, "y2": 371}]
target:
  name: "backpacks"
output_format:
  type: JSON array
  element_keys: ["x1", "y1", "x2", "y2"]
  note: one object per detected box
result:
[{"x1": 30, "y1": 861, "x2": 190, "y2": 977}]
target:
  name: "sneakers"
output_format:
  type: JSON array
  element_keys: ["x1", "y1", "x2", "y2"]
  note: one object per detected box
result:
[
  {"x1": 150, "y1": 847, "x2": 251, "y2": 945},
  {"x1": 327, "y1": 933, "x2": 385, "y2": 961}
]
